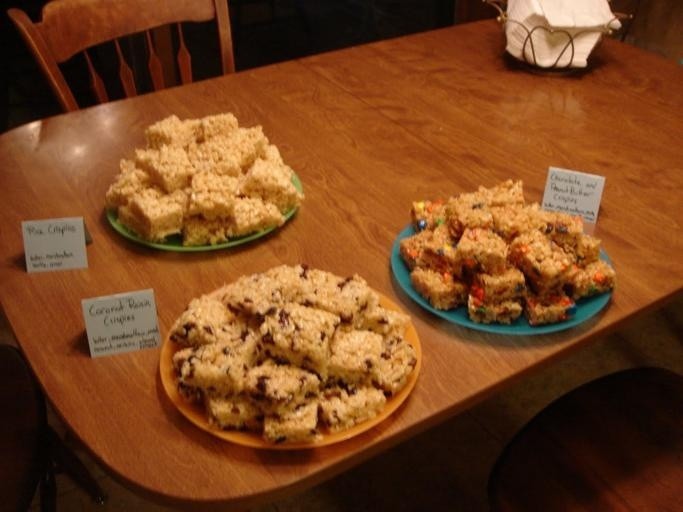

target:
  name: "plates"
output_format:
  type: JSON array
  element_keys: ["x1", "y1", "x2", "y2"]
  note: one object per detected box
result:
[
  {"x1": 388, "y1": 220, "x2": 618, "y2": 338},
  {"x1": 157, "y1": 274, "x2": 425, "y2": 454},
  {"x1": 103, "y1": 165, "x2": 305, "y2": 256}
]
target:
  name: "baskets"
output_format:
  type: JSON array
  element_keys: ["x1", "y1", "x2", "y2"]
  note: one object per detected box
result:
[{"x1": 497, "y1": 9, "x2": 633, "y2": 73}]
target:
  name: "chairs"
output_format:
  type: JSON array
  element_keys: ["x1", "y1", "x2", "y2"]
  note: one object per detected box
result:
[
  {"x1": 3, "y1": 343, "x2": 107, "y2": 509},
  {"x1": 484, "y1": 366, "x2": 682, "y2": 511},
  {"x1": 6, "y1": 2, "x2": 238, "y2": 113}
]
